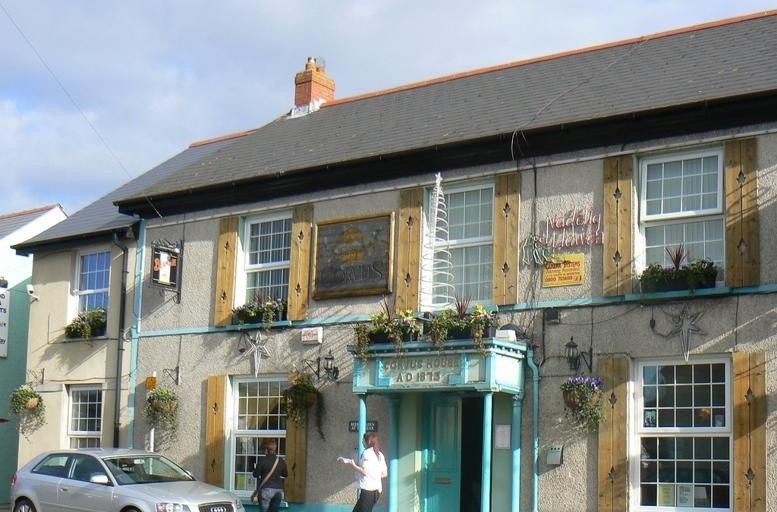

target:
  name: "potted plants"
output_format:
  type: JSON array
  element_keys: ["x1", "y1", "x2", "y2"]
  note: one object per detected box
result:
[
  {"x1": 640, "y1": 243, "x2": 718, "y2": 292},
  {"x1": 65, "y1": 306, "x2": 106, "y2": 342},
  {"x1": 231, "y1": 289, "x2": 274, "y2": 331},
  {"x1": 431, "y1": 293, "x2": 498, "y2": 359},
  {"x1": 282, "y1": 371, "x2": 327, "y2": 442},
  {"x1": 143, "y1": 387, "x2": 179, "y2": 447},
  {"x1": 352, "y1": 295, "x2": 419, "y2": 369}
]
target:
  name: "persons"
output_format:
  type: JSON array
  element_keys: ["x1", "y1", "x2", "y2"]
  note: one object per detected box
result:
[
  {"x1": 253, "y1": 440, "x2": 288, "y2": 512},
  {"x1": 339, "y1": 431, "x2": 388, "y2": 512}
]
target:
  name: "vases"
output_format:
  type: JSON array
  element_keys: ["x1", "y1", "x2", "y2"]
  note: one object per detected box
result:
[
  {"x1": 275, "y1": 310, "x2": 287, "y2": 320},
  {"x1": 563, "y1": 389, "x2": 601, "y2": 411},
  {"x1": 24, "y1": 397, "x2": 39, "y2": 410}
]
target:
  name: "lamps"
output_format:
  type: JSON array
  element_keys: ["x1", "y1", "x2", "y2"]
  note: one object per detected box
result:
[
  {"x1": 324, "y1": 349, "x2": 339, "y2": 379},
  {"x1": 566, "y1": 336, "x2": 581, "y2": 372}
]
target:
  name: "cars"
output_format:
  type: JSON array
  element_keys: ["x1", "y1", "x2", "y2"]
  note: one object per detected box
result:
[{"x1": 10, "y1": 446, "x2": 247, "y2": 511}]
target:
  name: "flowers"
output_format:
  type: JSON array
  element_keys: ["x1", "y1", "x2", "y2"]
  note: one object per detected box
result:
[
  {"x1": 271, "y1": 296, "x2": 287, "y2": 311},
  {"x1": 7, "y1": 383, "x2": 44, "y2": 437},
  {"x1": 561, "y1": 372, "x2": 605, "y2": 431}
]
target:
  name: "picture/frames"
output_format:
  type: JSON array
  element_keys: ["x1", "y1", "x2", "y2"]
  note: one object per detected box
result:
[{"x1": 311, "y1": 210, "x2": 396, "y2": 302}]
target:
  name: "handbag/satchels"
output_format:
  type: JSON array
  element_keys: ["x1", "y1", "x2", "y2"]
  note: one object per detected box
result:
[{"x1": 251, "y1": 489, "x2": 260, "y2": 505}]
